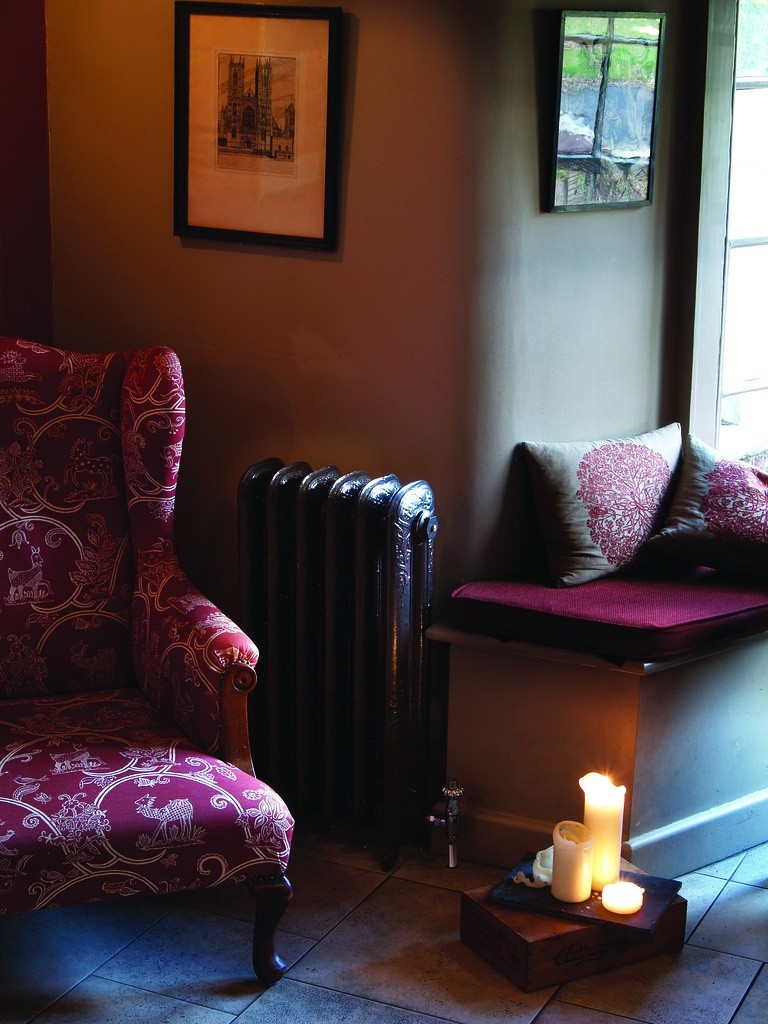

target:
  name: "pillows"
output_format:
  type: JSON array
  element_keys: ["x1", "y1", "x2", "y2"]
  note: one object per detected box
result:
[
  {"x1": 520, "y1": 422, "x2": 683, "y2": 584},
  {"x1": 647, "y1": 434, "x2": 768, "y2": 586}
]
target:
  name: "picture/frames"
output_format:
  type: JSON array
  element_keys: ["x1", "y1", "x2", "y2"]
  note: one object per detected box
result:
[{"x1": 173, "y1": 0, "x2": 343, "y2": 251}]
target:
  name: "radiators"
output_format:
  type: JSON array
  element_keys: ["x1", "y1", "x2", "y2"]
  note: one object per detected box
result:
[{"x1": 235, "y1": 458, "x2": 436, "y2": 871}]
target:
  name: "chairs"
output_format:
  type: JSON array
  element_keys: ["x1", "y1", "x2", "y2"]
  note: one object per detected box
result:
[{"x1": 0, "y1": 337, "x2": 297, "y2": 979}]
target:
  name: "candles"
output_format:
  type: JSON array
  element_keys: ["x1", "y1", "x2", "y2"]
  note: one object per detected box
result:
[{"x1": 517, "y1": 771, "x2": 645, "y2": 914}]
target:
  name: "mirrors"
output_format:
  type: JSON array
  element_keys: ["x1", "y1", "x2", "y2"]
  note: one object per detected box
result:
[{"x1": 548, "y1": 11, "x2": 668, "y2": 215}]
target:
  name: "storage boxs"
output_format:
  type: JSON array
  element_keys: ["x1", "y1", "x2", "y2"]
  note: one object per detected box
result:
[{"x1": 460, "y1": 886, "x2": 686, "y2": 992}]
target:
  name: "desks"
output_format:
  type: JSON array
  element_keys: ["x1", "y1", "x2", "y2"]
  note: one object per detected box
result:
[{"x1": 424, "y1": 570, "x2": 768, "y2": 882}]
